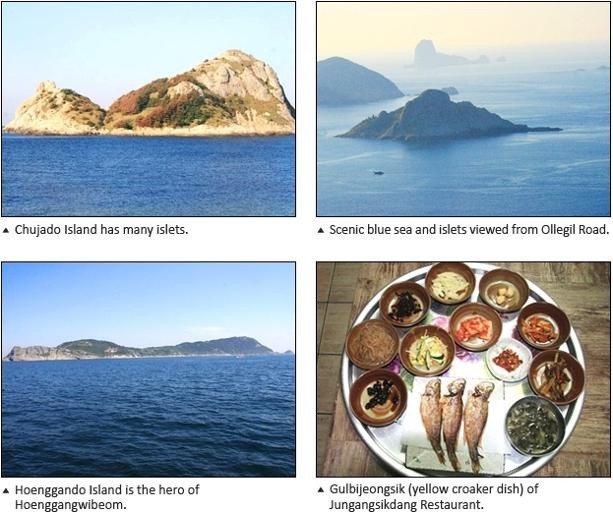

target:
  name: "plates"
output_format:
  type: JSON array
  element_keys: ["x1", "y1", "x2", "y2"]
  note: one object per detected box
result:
[{"x1": 346, "y1": 261, "x2": 585, "y2": 458}]
